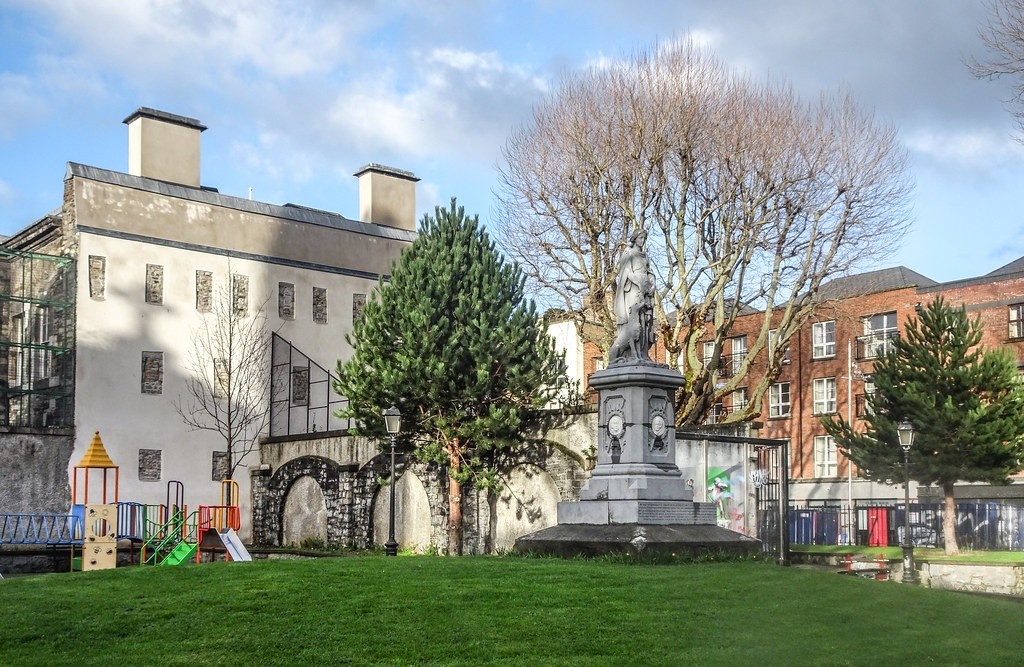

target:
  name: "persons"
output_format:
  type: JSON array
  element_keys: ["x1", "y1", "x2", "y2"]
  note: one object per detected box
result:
[{"x1": 708, "y1": 477, "x2": 728, "y2": 520}]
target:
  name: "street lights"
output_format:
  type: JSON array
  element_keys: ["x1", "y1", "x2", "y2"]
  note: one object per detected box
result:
[{"x1": 895, "y1": 415, "x2": 920, "y2": 586}]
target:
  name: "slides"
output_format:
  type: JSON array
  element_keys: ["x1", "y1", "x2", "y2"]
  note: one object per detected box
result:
[{"x1": 211, "y1": 528, "x2": 252, "y2": 561}]
